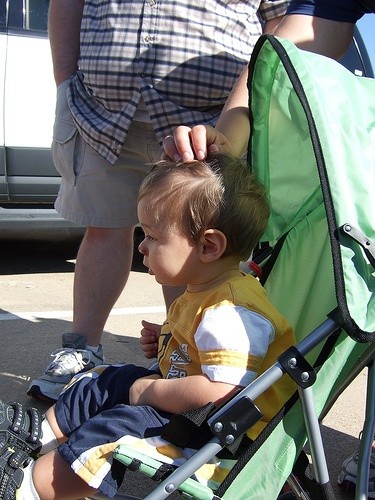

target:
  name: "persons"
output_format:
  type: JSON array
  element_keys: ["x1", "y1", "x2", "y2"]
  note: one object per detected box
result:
[
  {"x1": 25, "y1": 0, "x2": 291, "y2": 399},
  {"x1": 161, "y1": 0, "x2": 375, "y2": 500},
  {"x1": 0, "y1": 150, "x2": 300, "y2": 500}
]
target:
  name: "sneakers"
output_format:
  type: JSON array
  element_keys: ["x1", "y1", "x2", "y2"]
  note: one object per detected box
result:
[{"x1": 26, "y1": 332, "x2": 107, "y2": 403}]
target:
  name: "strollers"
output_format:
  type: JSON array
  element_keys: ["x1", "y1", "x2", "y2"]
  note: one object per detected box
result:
[{"x1": 112, "y1": 35, "x2": 375, "y2": 500}]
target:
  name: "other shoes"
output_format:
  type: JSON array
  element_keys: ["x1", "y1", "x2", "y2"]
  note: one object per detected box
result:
[
  {"x1": 0, "y1": 399, "x2": 43, "y2": 461},
  {"x1": 0, "y1": 436, "x2": 33, "y2": 500}
]
